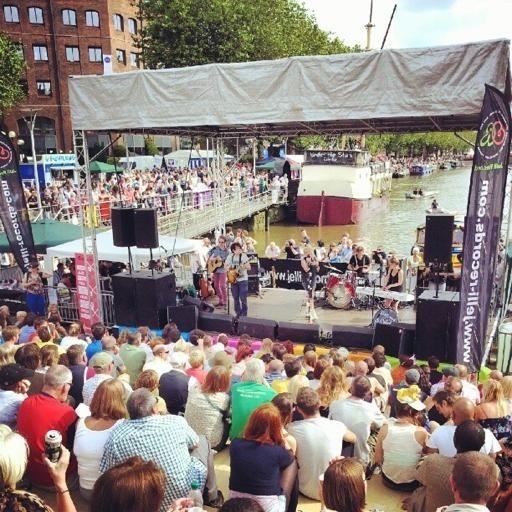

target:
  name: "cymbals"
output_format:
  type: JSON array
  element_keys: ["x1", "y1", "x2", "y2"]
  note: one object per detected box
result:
[{"x1": 324, "y1": 265, "x2": 343, "y2": 272}]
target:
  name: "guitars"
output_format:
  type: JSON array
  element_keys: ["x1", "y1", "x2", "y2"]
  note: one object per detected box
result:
[
  {"x1": 207, "y1": 256, "x2": 226, "y2": 274},
  {"x1": 227, "y1": 256, "x2": 255, "y2": 283}
]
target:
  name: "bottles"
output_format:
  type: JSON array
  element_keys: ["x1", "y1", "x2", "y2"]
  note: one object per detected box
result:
[{"x1": 186, "y1": 482, "x2": 204, "y2": 510}]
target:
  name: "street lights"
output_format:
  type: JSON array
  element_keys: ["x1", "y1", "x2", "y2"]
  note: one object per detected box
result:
[{"x1": 17, "y1": 106, "x2": 48, "y2": 220}]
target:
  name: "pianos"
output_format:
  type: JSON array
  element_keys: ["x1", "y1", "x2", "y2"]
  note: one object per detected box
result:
[{"x1": 357, "y1": 286, "x2": 413, "y2": 301}]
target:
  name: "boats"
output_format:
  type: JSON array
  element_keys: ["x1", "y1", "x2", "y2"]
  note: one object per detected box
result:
[
  {"x1": 404, "y1": 188, "x2": 440, "y2": 199},
  {"x1": 294, "y1": 133, "x2": 392, "y2": 227},
  {"x1": 424, "y1": 205, "x2": 448, "y2": 212},
  {"x1": 410, "y1": 211, "x2": 465, "y2": 278},
  {"x1": 392, "y1": 156, "x2": 464, "y2": 180}
]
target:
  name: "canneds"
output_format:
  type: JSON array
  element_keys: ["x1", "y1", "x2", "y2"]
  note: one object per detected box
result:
[{"x1": 45, "y1": 429, "x2": 62, "y2": 464}]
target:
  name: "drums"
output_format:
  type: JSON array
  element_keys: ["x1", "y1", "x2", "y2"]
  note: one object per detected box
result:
[
  {"x1": 323, "y1": 274, "x2": 340, "y2": 290},
  {"x1": 345, "y1": 270, "x2": 358, "y2": 280},
  {"x1": 326, "y1": 282, "x2": 354, "y2": 309}
]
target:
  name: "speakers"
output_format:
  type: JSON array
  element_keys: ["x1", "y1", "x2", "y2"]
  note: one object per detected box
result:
[
  {"x1": 416, "y1": 290, "x2": 460, "y2": 364},
  {"x1": 332, "y1": 325, "x2": 375, "y2": 348},
  {"x1": 372, "y1": 323, "x2": 416, "y2": 359},
  {"x1": 112, "y1": 209, "x2": 136, "y2": 247},
  {"x1": 112, "y1": 271, "x2": 175, "y2": 330},
  {"x1": 424, "y1": 214, "x2": 454, "y2": 269},
  {"x1": 167, "y1": 305, "x2": 199, "y2": 332},
  {"x1": 197, "y1": 312, "x2": 234, "y2": 335},
  {"x1": 278, "y1": 321, "x2": 320, "y2": 343},
  {"x1": 136, "y1": 207, "x2": 159, "y2": 248},
  {"x1": 237, "y1": 316, "x2": 276, "y2": 339}
]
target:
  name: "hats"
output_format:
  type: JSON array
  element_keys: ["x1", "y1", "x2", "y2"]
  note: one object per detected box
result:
[
  {"x1": 335, "y1": 345, "x2": 469, "y2": 416},
  {"x1": 170, "y1": 350, "x2": 190, "y2": 368},
  {"x1": 91, "y1": 351, "x2": 114, "y2": 368},
  {"x1": 0, "y1": 362, "x2": 34, "y2": 390}
]
